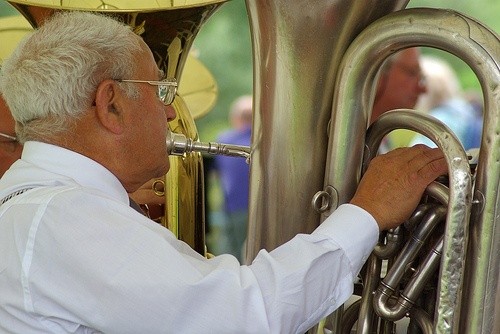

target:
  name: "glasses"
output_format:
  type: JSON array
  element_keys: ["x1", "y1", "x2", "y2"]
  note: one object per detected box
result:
[{"x1": 91, "y1": 76, "x2": 179, "y2": 106}]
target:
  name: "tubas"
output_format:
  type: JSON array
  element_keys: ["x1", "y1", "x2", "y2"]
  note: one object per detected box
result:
[{"x1": 3, "y1": 0, "x2": 498, "y2": 334}]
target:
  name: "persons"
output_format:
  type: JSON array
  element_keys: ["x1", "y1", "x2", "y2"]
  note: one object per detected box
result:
[
  {"x1": 215, "y1": 39, "x2": 484, "y2": 264},
  {"x1": 0, "y1": 7, "x2": 449, "y2": 333}
]
